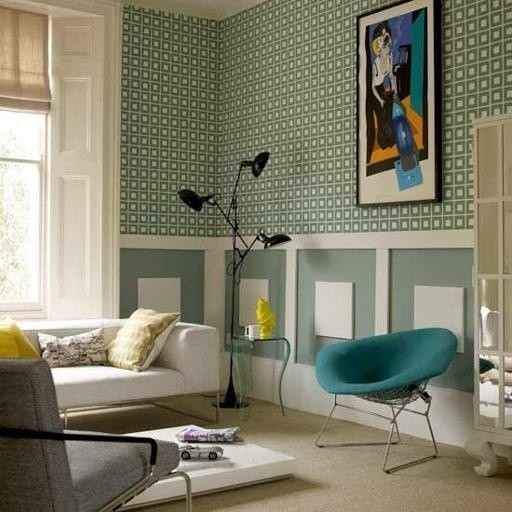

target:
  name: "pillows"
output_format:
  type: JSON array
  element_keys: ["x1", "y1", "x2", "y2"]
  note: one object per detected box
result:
[
  {"x1": 107, "y1": 308, "x2": 182, "y2": 371},
  {"x1": 0, "y1": 320, "x2": 41, "y2": 360},
  {"x1": 37, "y1": 328, "x2": 108, "y2": 368}
]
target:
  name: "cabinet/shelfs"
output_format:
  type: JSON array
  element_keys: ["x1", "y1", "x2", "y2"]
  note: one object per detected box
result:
[{"x1": 465, "y1": 114, "x2": 512, "y2": 477}]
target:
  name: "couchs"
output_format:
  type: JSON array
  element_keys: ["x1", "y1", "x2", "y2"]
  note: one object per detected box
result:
[{"x1": 0, "y1": 320, "x2": 220, "y2": 429}]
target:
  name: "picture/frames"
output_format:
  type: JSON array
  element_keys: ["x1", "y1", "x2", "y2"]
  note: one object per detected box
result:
[{"x1": 356, "y1": 0, "x2": 442, "y2": 206}]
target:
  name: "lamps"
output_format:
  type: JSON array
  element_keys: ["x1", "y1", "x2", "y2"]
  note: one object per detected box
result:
[{"x1": 179, "y1": 151, "x2": 291, "y2": 411}]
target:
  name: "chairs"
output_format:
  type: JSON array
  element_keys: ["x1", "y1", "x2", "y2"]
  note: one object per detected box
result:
[
  {"x1": 0, "y1": 358, "x2": 192, "y2": 512},
  {"x1": 315, "y1": 327, "x2": 458, "y2": 474}
]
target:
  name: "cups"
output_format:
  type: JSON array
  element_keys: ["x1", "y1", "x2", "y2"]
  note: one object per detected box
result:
[{"x1": 243, "y1": 325, "x2": 261, "y2": 341}]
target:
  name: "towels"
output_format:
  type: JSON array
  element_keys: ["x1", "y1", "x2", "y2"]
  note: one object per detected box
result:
[{"x1": 176, "y1": 426, "x2": 240, "y2": 442}]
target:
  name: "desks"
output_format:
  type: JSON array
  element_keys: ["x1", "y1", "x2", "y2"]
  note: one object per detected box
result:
[{"x1": 231, "y1": 336, "x2": 290, "y2": 420}]
